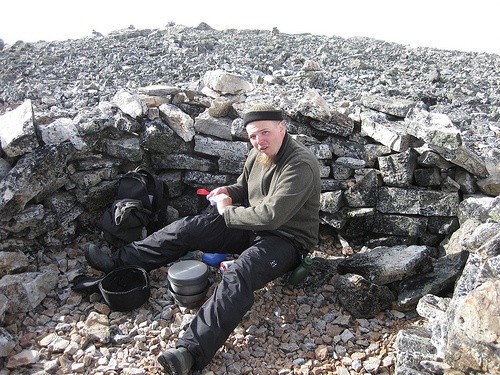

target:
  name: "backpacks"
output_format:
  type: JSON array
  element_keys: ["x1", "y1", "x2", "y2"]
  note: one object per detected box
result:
[{"x1": 99, "y1": 166, "x2": 168, "y2": 248}]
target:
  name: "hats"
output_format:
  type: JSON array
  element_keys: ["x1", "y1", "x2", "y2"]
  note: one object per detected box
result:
[{"x1": 243, "y1": 103, "x2": 283, "y2": 128}]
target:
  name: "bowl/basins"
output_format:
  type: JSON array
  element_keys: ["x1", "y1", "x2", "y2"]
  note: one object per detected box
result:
[
  {"x1": 98, "y1": 267, "x2": 150, "y2": 310},
  {"x1": 167, "y1": 283, "x2": 209, "y2": 304},
  {"x1": 167, "y1": 260, "x2": 208, "y2": 295}
]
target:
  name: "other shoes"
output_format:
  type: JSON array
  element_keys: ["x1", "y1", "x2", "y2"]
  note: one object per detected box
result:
[
  {"x1": 84, "y1": 243, "x2": 118, "y2": 274},
  {"x1": 156, "y1": 347, "x2": 193, "y2": 375}
]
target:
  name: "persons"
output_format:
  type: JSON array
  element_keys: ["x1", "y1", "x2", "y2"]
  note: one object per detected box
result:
[{"x1": 83, "y1": 103, "x2": 321, "y2": 375}]
